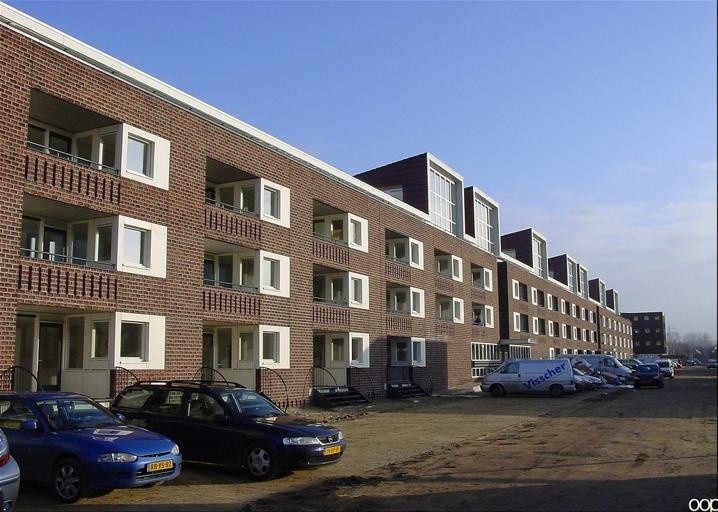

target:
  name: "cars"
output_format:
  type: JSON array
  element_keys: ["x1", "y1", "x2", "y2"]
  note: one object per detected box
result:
[
  {"x1": 707, "y1": 359, "x2": 717, "y2": 368},
  {"x1": 633, "y1": 359, "x2": 681, "y2": 390},
  {"x1": 110, "y1": 379, "x2": 346, "y2": 481},
  {"x1": 1, "y1": 391, "x2": 182, "y2": 512},
  {"x1": 572, "y1": 361, "x2": 620, "y2": 391}
]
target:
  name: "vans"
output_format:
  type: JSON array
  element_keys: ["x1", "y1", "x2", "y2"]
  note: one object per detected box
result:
[
  {"x1": 480, "y1": 359, "x2": 577, "y2": 398},
  {"x1": 558, "y1": 354, "x2": 633, "y2": 380}
]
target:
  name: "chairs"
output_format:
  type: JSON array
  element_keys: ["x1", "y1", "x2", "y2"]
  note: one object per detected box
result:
[
  {"x1": 190, "y1": 399, "x2": 209, "y2": 419},
  {"x1": 39, "y1": 403, "x2": 55, "y2": 422}
]
